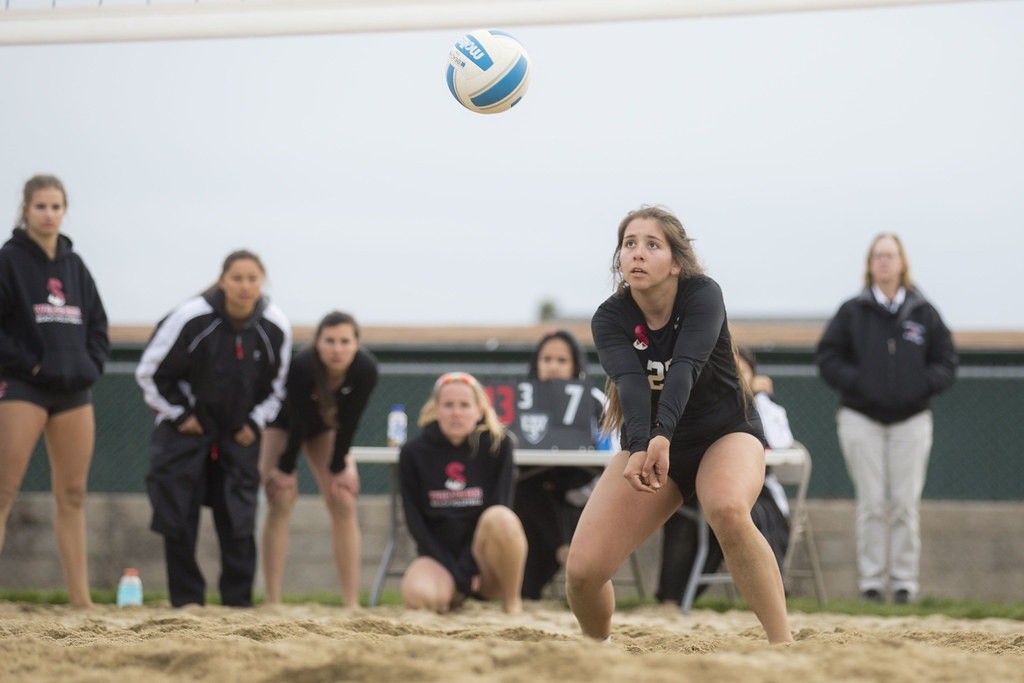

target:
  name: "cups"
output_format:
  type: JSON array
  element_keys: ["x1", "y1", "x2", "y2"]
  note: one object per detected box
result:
[{"x1": 593, "y1": 431, "x2": 611, "y2": 450}]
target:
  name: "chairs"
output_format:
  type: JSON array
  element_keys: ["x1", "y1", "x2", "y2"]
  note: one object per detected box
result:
[
  {"x1": 682, "y1": 438, "x2": 829, "y2": 611},
  {"x1": 548, "y1": 550, "x2": 648, "y2": 601}
]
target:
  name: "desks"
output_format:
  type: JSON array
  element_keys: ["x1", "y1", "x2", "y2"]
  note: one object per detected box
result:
[{"x1": 348, "y1": 446, "x2": 805, "y2": 613}]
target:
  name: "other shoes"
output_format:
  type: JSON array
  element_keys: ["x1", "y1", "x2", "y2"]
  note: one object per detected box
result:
[
  {"x1": 893, "y1": 581, "x2": 915, "y2": 605},
  {"x1": 856, "y1": 577, "x2": 886, "y2": 601}
]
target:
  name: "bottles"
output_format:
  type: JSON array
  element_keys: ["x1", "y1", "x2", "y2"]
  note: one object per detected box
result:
[
  {"x1": 117, "y1": 567, "x2": 143, "y2": 610},
  {"x1": 386, "y1": 404, "x2": 408, "y2": 449}
]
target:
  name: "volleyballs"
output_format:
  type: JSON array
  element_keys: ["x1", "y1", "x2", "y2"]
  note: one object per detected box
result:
[{"x1": 449, "y1": 30, "x2": 532, "y2": 116}]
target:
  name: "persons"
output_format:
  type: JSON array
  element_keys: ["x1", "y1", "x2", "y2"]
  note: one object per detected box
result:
[
  {"x1": 813, "y1": 230, "x2": 958, "y2": 608},
  {"x1": 132, "y1": 250, "x2": 292, "y2": 607},
  {"x1": 1, "y1": 173, "x2": 111, "y2": 618},
  {"x1": 399, "y1": 372, "x2": 529, "y2": 625},
  {"x1": 512, "y1": 331, "x2": 609, "y2": 602},
  {"x1": 272, "y1": 308, "x2": 377, "y2": 610},
  {"x1": 564, "y1": 207, "x2": 794, "y2": 651},
  {"x1": 649, "y1": 348, "x2": 803, "y2": 613}
]
